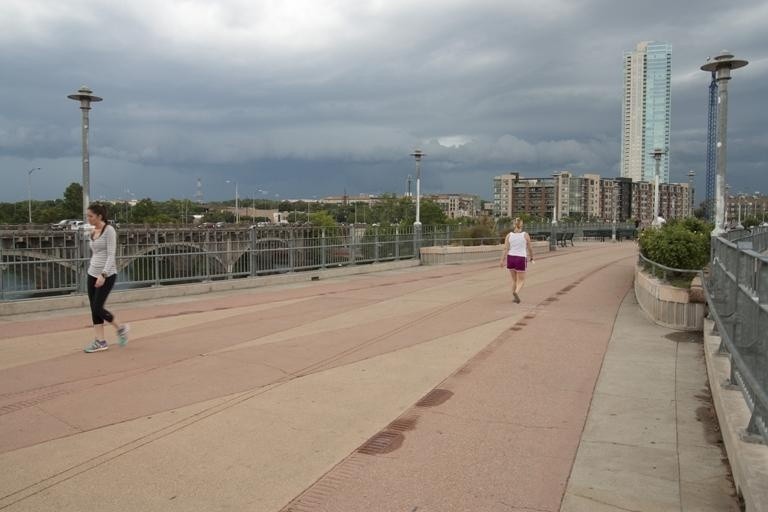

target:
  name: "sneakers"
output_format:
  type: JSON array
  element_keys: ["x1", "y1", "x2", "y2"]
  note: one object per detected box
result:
[
  {"x1": 115, "y1": 323, "x2": 130, "y2": 346},
  {"x1": 83, "y1": 339, "x2": 108, "y2": 353},
  {"x1": 513, "y1": 291, "x2": 520, "y2": 304}
]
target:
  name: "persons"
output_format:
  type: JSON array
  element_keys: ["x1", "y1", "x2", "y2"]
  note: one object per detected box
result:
[
  {"x1": 499, "y1": 217, "x2": 534, "y2": 303},
  {"x1": 633, "y1": 215, "x2": 644, "y2": 230},
  {"x1": 82, "y1": 203, "x2": 132, "y2": 354},
  {"x1": 651, "y1": 212, "x2": 667, "y2": 226}
]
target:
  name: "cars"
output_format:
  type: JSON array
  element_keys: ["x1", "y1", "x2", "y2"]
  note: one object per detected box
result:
[
  {"x1": 194, "y1": 221, "x2": 404, "y2": 231},
  {"x1": 50, "y1": 219, "x2": 121, "y2": 234}
]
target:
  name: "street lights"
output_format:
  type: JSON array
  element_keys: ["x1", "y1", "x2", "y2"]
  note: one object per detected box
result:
[
  {"x1": 648, "y1": 148, "x2": 666, "y2": 228},
  {"x1": 550, "y1": 170, "x2": 562, "y2": 252},
  {"x1": 469, "y1": 197, "x2": 472, "y2": 221},
  {"x1": 97, "y1": 187, "x2": 199, "y2": 225},
  {"x1": 608, "y1": 182, "x2": 621, "y2": 243},
  {"x1": 723, "y1": 181, "x2": 766, "y2": 234},
  {"x1": 223, "y1": 179, "x2": 365, "y2": 224},
  {"x1": 27, "y1": 165, "x2": 42, "y2": 227},
  {"x1": 408, "y1": 149, "x2": 429, "y2": 262},
  {"x1": 64, "y1": 84, "x2": 104, "y2": 299},
  {"x1": 699, "y1": 50, "x2": 750, "y2": 318},
  {"x1": 685, "y1": 168, "x2": 696, "y2": 221}
]
